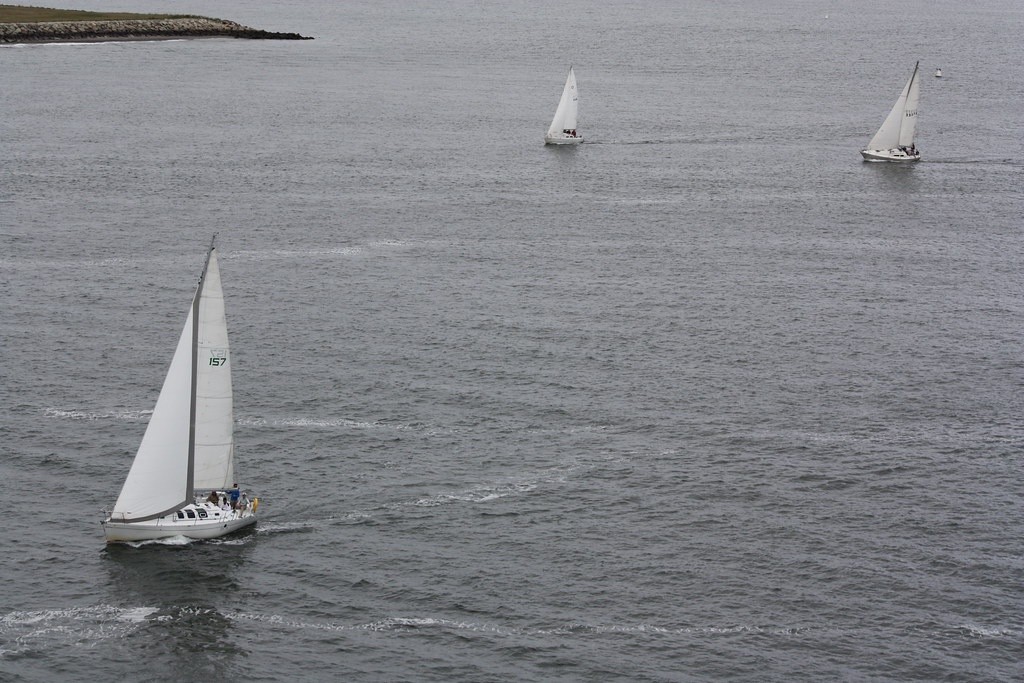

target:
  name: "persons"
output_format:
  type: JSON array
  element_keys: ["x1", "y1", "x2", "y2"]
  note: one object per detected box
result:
[
  {"x1": 902, "y1": 143, "x2": 919, "y2": 156},
  {"x1": 205, "y1": 483, "x2": 250, "y2": 518},
  {"x1": 563, "y1": 130, "x2": 576, "y2": 138}
]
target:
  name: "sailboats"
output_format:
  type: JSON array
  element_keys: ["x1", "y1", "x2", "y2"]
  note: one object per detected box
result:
[
  {"x1": 104, "y1": 236, "x2": 265, "y2": 544},
  {"x1": 541, "y1": 63, "x2": 585, "y2": 147},
  {"x1": 858, "y1": 57, "x2": 925, "y2": 166}
]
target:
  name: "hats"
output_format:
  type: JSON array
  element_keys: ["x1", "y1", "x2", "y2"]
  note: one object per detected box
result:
[
  {"x1": 223, "y1": 497, "x2": 227, "y2": 501},
  {"x1": 242, "y1": 493, "x2": 247, "y2": 496}
]
